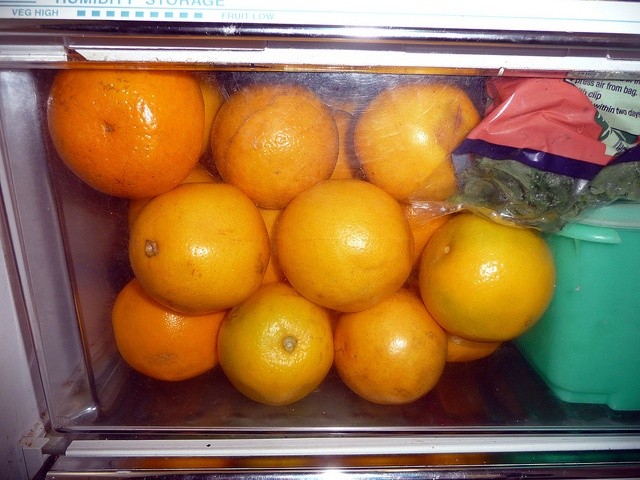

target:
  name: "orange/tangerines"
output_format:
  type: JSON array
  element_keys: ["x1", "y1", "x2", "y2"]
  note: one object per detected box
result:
[
  {"x1": 256, "y1": 204, "x2": 282, "y2": 287},
  {"x1": 216, "y1": 284, "x2": 331, "y2": 408},
  {"x1": 419, "y1": 212, "x2": 558, "y2": 343},
  {"x1": 448, "y1": 335, "x2": 501, "y2": 362},
  {"x1": 333, "y1": 292, "x2": 449, "y2": 403},
  {"x1": 47, "y1": 70, "x2": 206, "y2": 199},
  {"x1": 275, "y1": 181, "x2": 413, "y2": 315},
  {"x1": 128, "y1": 167, "x2": 216, "y2": 228},
  {"x1": 212, "y1": 86, "x2": 340, "y2": 208},
  {"x1": 354, "y1": 83, "x2": 488, "y2": 204},
  {"x1": 112, "y1": 273, "x2": 227, "y2": 382},
  {"x1": 327, "y1": 107, "x2": 367, "y2": 180}
]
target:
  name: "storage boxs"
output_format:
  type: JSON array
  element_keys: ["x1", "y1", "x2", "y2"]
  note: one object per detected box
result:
[{"x1": 511, "y1": 194, "x2": 640, "y2": 413}]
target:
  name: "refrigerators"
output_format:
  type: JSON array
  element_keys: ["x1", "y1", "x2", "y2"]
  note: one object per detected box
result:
[{"x1": 1, "y1": 0, "x2": 639, "y2": 478}]
